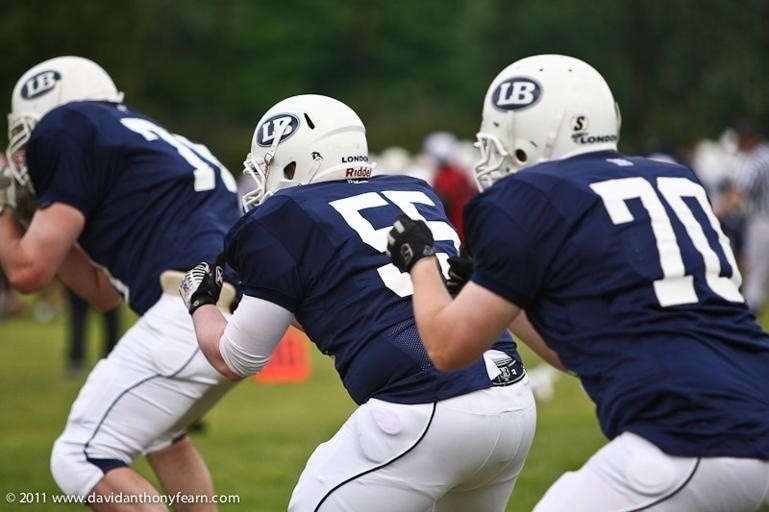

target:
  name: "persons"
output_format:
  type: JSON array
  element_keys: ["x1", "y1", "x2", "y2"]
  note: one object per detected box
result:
[
  {"x1": 0, "y1": 267, "x2": 25, "y2": 318},
  {"x1": 386, "y1": 52, "x2": 769, "y2": 512},
  {"x1": 175, "y1": 93, "x2": 537, "y2": 512},
  {"x1": 373, "y1": 129, "x2": 559, "y2": 400},
  {"x1": 66, "y1": 286, "x2": 120, "y2": 380},
  {"x1": 1, "y1": 55, "x2": 244, "y2": 512},
  {"x1": 674, "y1": 121, "x2": 769, "y2": 312}
]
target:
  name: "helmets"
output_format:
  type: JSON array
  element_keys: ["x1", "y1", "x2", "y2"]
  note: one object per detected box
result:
[
  {"x1": 242, "y1": 94, "x2": 375, "y2": 213},
  {"x1": 6, "y1": 56, "x2": 126, "y2": 193},
  {"x1": 474, "y1": 54, "x2": 621, "y2": 194}
]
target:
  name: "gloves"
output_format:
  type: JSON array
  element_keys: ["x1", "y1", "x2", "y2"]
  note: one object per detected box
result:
[
  {"x1": 387, "y1": 214, "x2": 436, "y2": 272},
  {"x1": 180, "y1": 260, "x2": 227, "y2": 313},
  {"x1": 1, "y1": 155, "x2": 17, "y2": 214},
  {"x1": 446, "y1": 245, "x2": 473, "y2": 298}
]
